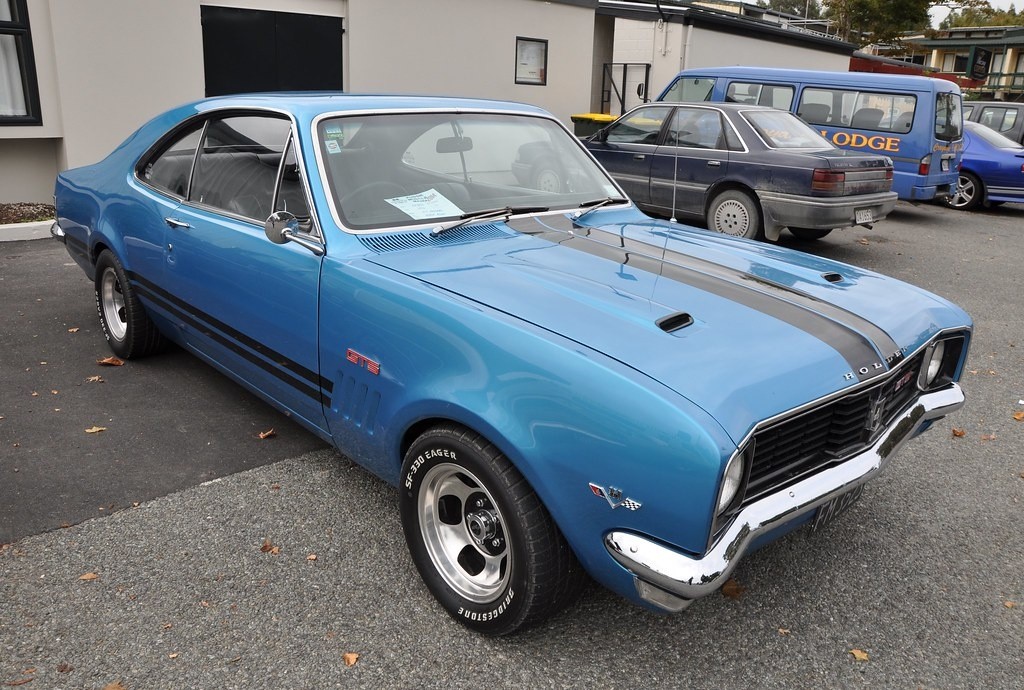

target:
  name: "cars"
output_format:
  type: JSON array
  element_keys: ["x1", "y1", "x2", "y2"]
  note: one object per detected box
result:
[
  {"x1": 512, "y1": 101, "x2": 898, "y2": 241},
  {"x1": 49, "y1": 91, "x2": 974, "y2": 639},
  {"x1": 877, "y1": 117, "x2": 1024, "y2": 211}
]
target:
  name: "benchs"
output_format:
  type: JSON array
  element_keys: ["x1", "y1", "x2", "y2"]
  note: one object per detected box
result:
[{"x1": 150, "y1": 148, "x2": 386, "y2": 222}]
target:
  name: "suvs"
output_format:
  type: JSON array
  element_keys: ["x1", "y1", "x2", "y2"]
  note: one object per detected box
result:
[{"x1": 939, "y1": 101, "x2": 1024, "y2": 146}]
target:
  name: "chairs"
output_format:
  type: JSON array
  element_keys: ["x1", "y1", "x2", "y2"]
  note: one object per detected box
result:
[
  {"x1": 801, "y1": 104, "x2": 831, "y2": 123},
  {"x1": 728, "y1": 84, "x2": 736, "y2": 98},
  {"x1": 675, "y1": 123, "x2": 701, "y2": 148},
  {"x1": 851, "y1": 108, "x2": 884, "y2": 129},
  {"x1": 892, "y1": 111, "x2": 914, "y2": 132},
  {"x1": 744, "y1": 84, "x2": 759, "y2": 104}
]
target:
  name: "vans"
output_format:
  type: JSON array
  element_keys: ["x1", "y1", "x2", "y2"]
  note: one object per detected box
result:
[{"x1": 636, "y1": 66, "x2": 964, "y2": 201}]
